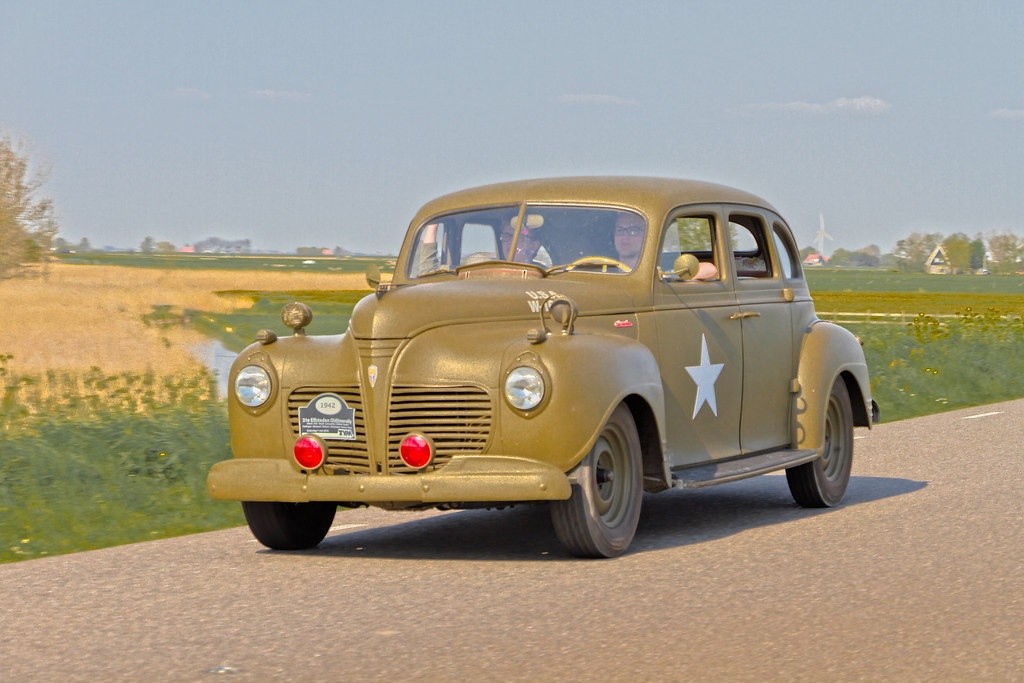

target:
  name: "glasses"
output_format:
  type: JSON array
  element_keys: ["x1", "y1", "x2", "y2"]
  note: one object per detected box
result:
[
  {"x1": 614, "y1": 226, "x2": 645, "y2": 237},
  {"x1": 499, "y1": 232, "x2": 536, "y2": 242}
]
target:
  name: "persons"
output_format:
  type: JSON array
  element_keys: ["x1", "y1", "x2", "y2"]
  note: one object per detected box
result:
[
  {"x1": 417, "y1": 218, "x2": 548, "y2": 275},
  {"x1": 607, "y1": 213, "x2": 718, "y2": 282}
]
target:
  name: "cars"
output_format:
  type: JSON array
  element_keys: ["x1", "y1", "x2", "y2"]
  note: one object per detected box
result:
[{"x1": 205, "y1": 174, "x2": 880, "y2": 561}]
